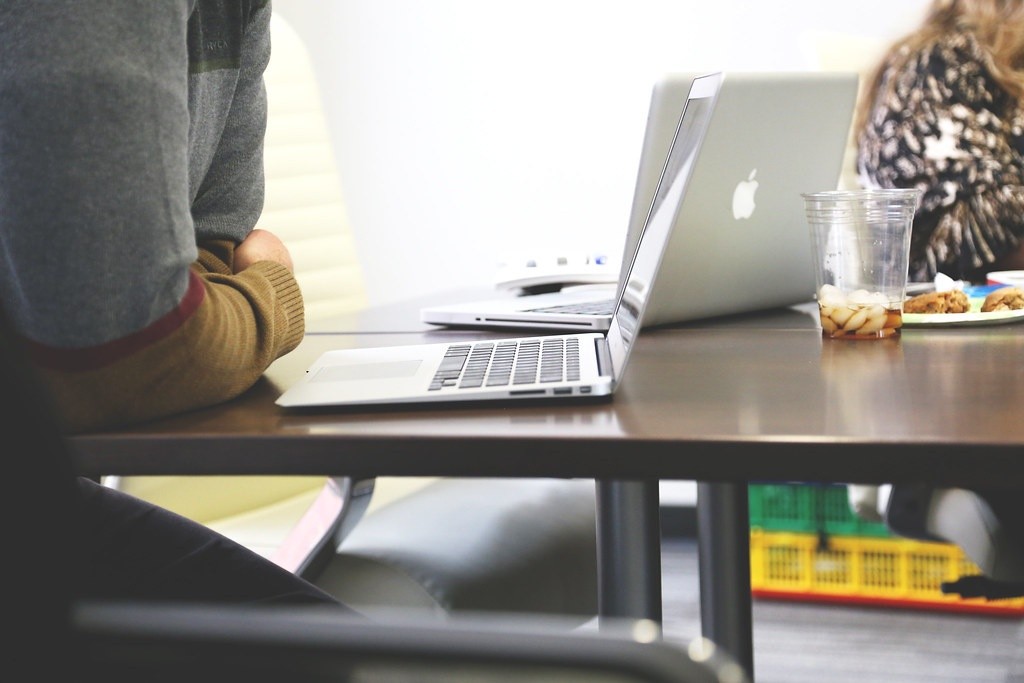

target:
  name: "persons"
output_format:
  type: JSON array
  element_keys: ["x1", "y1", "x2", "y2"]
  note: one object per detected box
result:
[
  {"x1": 855, "y1": 0, "x2": 1023, "y2": 583},
  {"x1": 0, "y1": 0, "x2": 372, "y2": 682}
]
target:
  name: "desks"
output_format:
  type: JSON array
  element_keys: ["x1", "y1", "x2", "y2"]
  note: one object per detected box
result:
[{"x1": 59, "y1": 269, "x2": 1024, "y2": 681}]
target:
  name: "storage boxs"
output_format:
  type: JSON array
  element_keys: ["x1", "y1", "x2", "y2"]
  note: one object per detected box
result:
[{"x1": 748, "y1": 484, "x2": 1024, "y2": 618}]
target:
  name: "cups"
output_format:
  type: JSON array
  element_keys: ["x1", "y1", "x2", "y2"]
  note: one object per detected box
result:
[{"x1": 800, "y1": 189, "x2": 923, "y2": 341}]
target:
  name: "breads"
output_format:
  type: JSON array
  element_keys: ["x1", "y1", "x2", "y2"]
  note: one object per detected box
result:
[
  {"x1": 903, "y1": 290, "x2": 970, "y2": 314},
  {"x1": 980, "y1": 287, "x2": 1024, "y2": 312}
]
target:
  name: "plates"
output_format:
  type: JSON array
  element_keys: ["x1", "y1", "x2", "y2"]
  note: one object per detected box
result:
[{"x1": 901, "y1": 284, "x2": 1024, "y2": 327}]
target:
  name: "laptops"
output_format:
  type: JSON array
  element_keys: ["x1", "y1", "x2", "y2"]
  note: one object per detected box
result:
[
  {"x1": 272, "y1": 72, "x2": 727, "y2": 408},
  {"x1": 422, "y1": 70, "x2": 863, "y2": 331}
]
